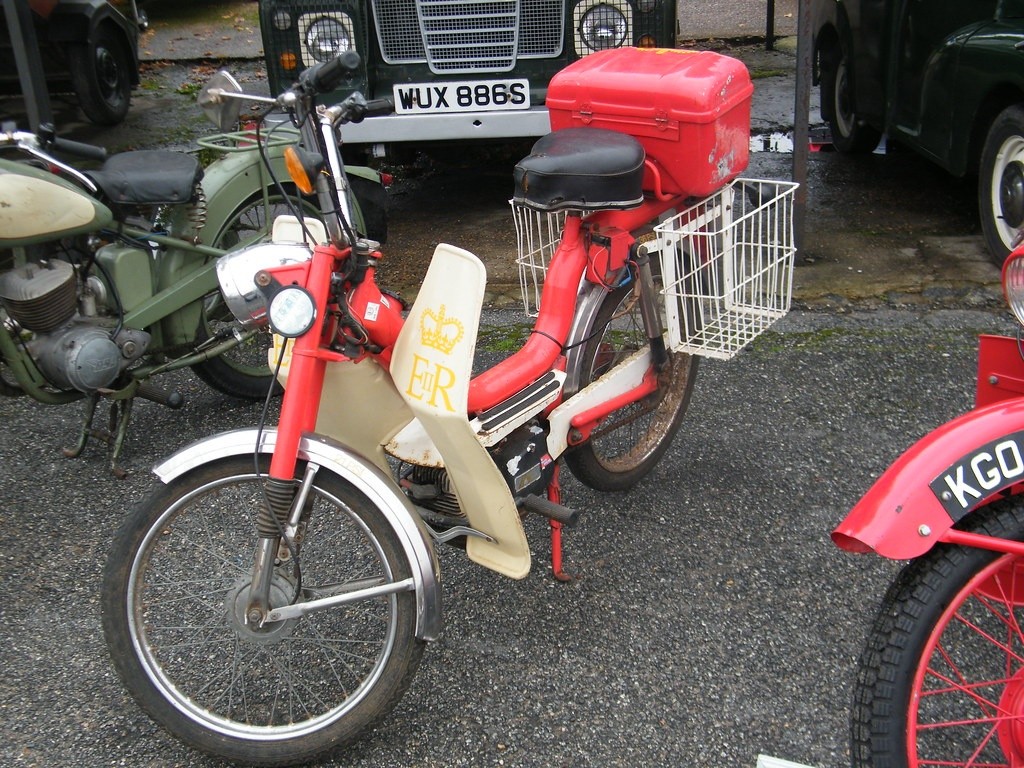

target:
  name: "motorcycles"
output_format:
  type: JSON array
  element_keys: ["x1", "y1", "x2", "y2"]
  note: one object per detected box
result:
[{"x1": 0, "y1": 69, "x2": 394, "y2": 479}]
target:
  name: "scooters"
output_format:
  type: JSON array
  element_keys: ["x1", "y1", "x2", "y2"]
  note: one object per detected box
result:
[
  {"x1": 830, "y1": 241, "x2": 1024, "y2": 768},
  {"x1": 99, "y1": 51, "x2": 799, "y2": 768}
]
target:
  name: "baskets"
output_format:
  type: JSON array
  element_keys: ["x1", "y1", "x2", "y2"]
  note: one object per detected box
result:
[
  {"x1": 652, "y1": 178, "x2": 801, "y2": 361},
  {"x1": 509, "y1": 195, "x2": 595, "y2": 319}
]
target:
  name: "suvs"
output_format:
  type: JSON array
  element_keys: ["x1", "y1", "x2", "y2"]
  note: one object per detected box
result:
[{"x1": 257, "y1": 0, "x2": 675, "y2": 245}]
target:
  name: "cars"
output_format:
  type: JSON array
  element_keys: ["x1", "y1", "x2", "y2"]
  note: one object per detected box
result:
[{"x1": 820, "y1": 2, "x2": 1023, "y2": 268}]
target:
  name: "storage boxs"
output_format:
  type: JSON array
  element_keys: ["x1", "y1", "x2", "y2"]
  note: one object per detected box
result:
[{"x1": 544, "y1": 45, "x2": 755, "y2": 197}]
